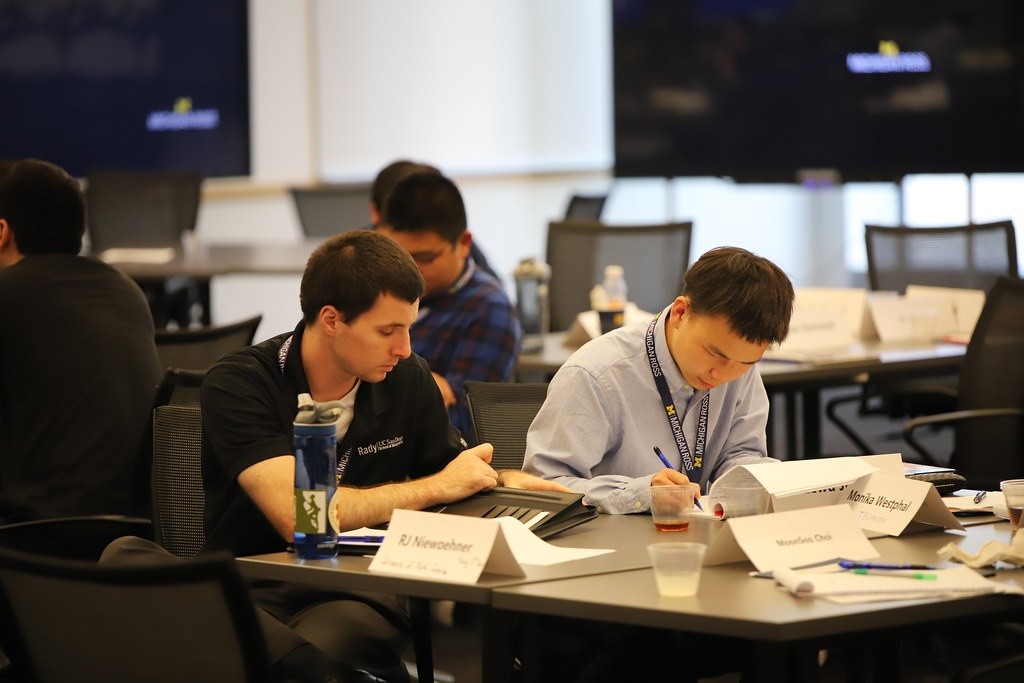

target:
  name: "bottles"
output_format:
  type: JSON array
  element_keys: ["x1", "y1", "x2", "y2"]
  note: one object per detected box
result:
[
  {"x1": 292, "y1": 392, "x2": 340, "y2": 559},
  {"x1": 514, "y1": 257, "x2": 552, "y2": 354},
  {"x1": 597, "y1": 265, "x2": 627, "y2": 335}
]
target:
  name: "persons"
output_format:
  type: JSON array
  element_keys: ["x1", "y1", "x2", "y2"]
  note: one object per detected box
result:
[
  {"x1": 98, "y1": 160, "x2": 517, "y2": 683},
  {"x1": 0, "y1": 157, "x2": 161, "y2": 557},
  {"x1": 524, "y1": 246, "x2": 800, "y2": 683}
]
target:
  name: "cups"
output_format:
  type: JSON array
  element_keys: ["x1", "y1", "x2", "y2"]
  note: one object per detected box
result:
[
  {"x1": 646, "y1": 542, "x2": 706, "y2": 597},
  {"x1": 648, "y1": 484, "x2": 697, "y2": 533},
  {"x1": 1000, "y1": 479, "x2": 1024, "y2": 531}
]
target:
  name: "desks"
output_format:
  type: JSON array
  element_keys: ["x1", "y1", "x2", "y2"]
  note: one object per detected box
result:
[
  {"x1": 516, "y1": 321, "x2": 967, "y2": 458},
  {"x1": 233, "y1": 489, "x2": 724, "y2": 683},
  {"x1": 490, "y1": 515, "x2": 1024, "y2": 683}
]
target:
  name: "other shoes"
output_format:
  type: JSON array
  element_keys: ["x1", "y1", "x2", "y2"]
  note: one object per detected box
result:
[{"x1": 321, "y1": 669, "x2": 390, "y2": 683}]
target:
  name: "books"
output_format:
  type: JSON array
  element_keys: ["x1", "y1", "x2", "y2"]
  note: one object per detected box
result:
[{"x1": 686, "y1": 457, "x2": 881, "y2": 522}]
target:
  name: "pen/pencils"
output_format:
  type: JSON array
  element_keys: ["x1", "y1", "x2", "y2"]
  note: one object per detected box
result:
[
  {"x1": 653, "y1": 447, "x2": 704, "y2": 512},
  {"x1": 974, "y1": 490, "x2": 986, "y2": 503},
  {"x1": 849, "y1": 569, "x2": 937, "y2": 581},
  {"x1": 336, "y1": 536, "x2": 384, "y2": 542},
  {"x1": 838, "y1": 561, "x2": 938, "y2": 570}
]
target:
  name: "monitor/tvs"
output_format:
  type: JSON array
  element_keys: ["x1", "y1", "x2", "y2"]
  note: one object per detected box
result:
[
  {"x1": 0, "y1": 0, "x2": 251, "y2": 180},
  {"x1": 611, "y1": 0, "x2": 1024, "y2": 184}
]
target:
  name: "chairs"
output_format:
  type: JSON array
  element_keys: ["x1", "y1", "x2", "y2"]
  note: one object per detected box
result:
[
  {"x1": 0, "y1": 314, "x2": 436, "y2": 683},
  {"x1": 540, "y1": 221, "x2": 695, "y2": 333},
  {"x1": 827, "y1": 221, "x2": 1024, "y2": 485}
]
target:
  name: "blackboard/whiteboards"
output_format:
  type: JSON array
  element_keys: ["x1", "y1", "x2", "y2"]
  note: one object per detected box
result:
[{"x1": 201, "y1": 0, "x2": 617, "y2": 199}]
